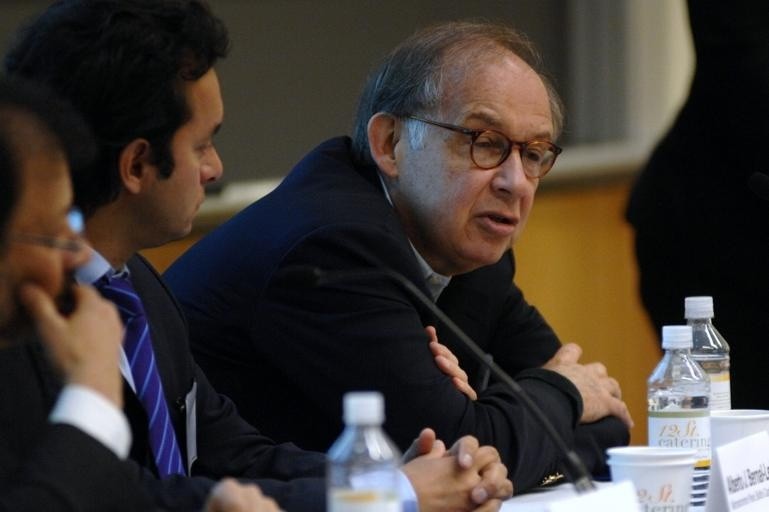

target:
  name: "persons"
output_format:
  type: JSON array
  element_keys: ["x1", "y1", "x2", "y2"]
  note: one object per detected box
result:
[
  {"x1": 1, "y1": 0, "x2": 515, "y2": 510},
  {"x1": 158, "y1": 20, "x2": 634, "y2": 498},
  {"x1": 0, "y1": 80, "x2": 282, "y2": 510},
  {"x1": 621, "y1": 0, "x2": 767, "y2": 412}
]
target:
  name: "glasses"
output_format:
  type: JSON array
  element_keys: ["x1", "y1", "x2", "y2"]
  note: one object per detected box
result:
[{"x1": 392, "y1": 110, "x2": 564, "y2": 180}]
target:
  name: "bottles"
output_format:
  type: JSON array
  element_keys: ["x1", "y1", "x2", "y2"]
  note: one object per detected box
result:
[
  {"x1": 646, "y1": 324, "x2": 711, "y2": 460},
  {"x1": 328, "y1": 389, "x2": 404, "y2": 510},
  {"x1": 681, "y1": 293, "x2": 734, "y2": 412}
]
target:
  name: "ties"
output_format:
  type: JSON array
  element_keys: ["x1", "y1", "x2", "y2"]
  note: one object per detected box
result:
[{"x1": 96, "y1": 275, "x2": 189, "y2": 482}]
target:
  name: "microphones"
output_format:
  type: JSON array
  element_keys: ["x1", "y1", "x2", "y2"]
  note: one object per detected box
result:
[{"x1": 316, "y1": 234, "x2": 592, "y2": 494}]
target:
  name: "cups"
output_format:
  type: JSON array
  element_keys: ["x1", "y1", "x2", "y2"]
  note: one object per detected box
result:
[
  {"x1": 709, "y1": 407, "x2": 768, "y2": 512},
  {"x1": 607, "y1": 444, "x2": 698, "y2": 511}
]
